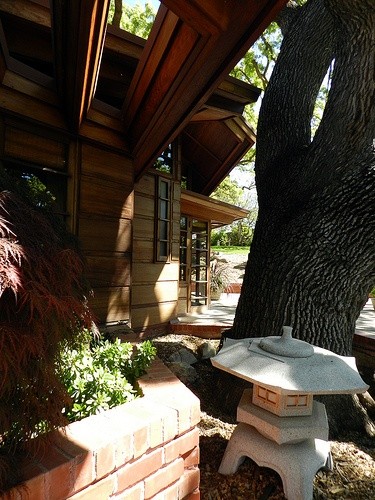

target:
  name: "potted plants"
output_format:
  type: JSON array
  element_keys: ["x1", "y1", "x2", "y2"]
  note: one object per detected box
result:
[{"x1": 200, "y1": 258, "x2": 231, "y2": 300}]
